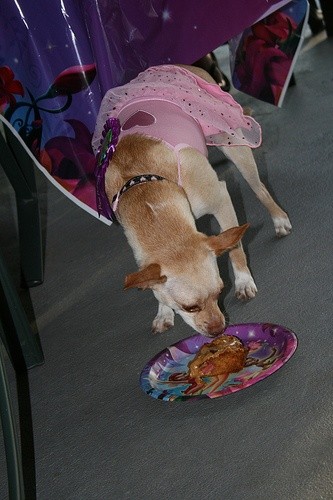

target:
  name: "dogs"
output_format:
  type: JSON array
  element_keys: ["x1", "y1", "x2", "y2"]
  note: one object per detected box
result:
[{"x1": 91, "y1": 63, "x2": 293, "y2": 338}]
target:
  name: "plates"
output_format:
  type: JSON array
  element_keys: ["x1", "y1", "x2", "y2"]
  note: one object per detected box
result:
[{"x1": 138, "y1": 323, "x2": 298, "y2": 400}]
